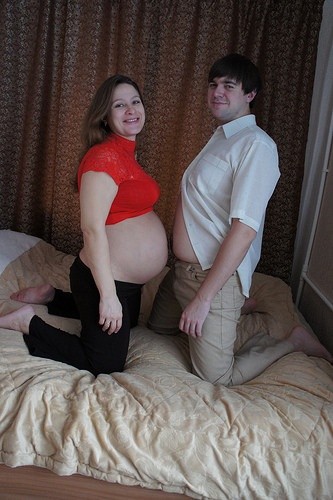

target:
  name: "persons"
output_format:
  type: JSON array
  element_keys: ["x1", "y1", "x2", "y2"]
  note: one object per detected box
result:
[
  {"x1": 148, "y1": 54, "x2": 333, "y2": 388},
  {"x1": 0, "y1": 74, "x2": 168, "y2": 375}
]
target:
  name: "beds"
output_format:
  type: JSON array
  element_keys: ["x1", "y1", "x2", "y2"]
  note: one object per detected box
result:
[{"x1": 0, "y1": 227, "x2": 333, "y2": 500}]
